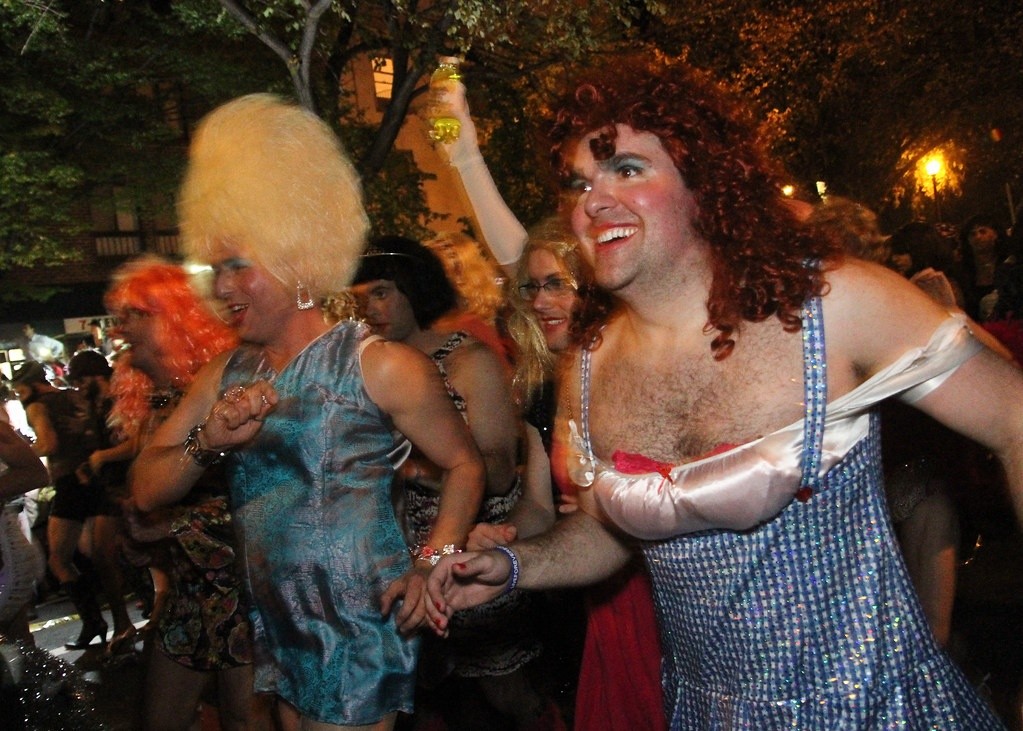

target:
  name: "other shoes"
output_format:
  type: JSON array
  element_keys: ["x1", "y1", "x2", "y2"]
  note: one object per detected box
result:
[{"x1": 136, "y1": 598, "x2": 146, "y2": 607}]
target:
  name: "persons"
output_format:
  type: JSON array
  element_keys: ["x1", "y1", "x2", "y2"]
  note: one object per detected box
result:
[
  {"x1": 0, "y1": 261, "x2": 275, "y2": 731},
  {"x1": 806, "y1": 198, "x2": 1022, "y2": 672},
  {"x1": 135, "y1": 92, "x2": 483, "y2": 730},
  {"x1": 466, "y1": 216, "x2": 668, "y2": 730},
  {"x1": 352, "y1": 234, "x2": 565, "y2": 731},
  {"x1": 423, "y1": 40, "x2": 1023, "y2": 731}
]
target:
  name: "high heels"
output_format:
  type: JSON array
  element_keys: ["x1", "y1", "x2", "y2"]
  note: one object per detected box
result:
[
  {"x1": 64, "y1": 621, "x2": 107, "y2": 648},
  {"x1": 104, "y1": 624, "x2": 136, "y2": 652}
]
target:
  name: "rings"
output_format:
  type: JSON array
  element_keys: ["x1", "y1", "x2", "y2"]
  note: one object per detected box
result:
[{"x1": 225, "y1": 387, "x2": 243, "y2": 401}]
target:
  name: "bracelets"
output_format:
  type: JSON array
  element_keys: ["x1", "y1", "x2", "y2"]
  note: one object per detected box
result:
[
  {"x1": 497, "y1": 546, "x2": 518, "y2": 590},
  {"x1": 417, "y1": 545, "x2": 460, "y2": 565},
  {"x1": 185, "y1": 425, "x2": 222, "y2": 466}
]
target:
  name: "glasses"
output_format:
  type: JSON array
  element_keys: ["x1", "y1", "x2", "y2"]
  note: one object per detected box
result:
[{"x1": 517, "y1": 277, "x2": 572, "y2": 302}]
